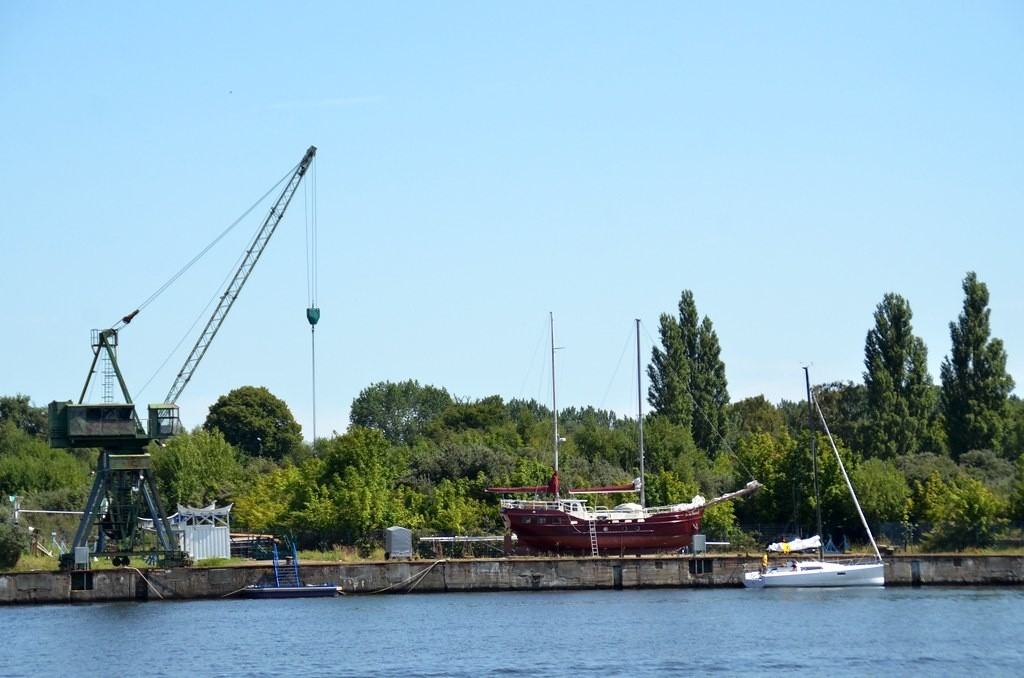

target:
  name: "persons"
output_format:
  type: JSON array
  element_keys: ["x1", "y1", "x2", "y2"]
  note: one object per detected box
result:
[
  {"x1": 762, "y1": 550, "x2": 769, "y2": 573},
  {"x1": 791, "y1": 559, "x2": 799, "y2": 571}
]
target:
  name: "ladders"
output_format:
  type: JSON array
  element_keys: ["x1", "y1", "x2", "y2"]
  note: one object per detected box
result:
[{"x1": 588, "y1": 513, "x2": 599, "y2": 555}]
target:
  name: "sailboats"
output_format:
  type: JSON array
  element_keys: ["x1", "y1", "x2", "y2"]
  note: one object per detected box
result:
[
  {"x1": 486, "y1": 310, "x2": 765, "y2": 555},
  {"x1": 740, "y1": 366, "x2": 886, "y2": 588}
]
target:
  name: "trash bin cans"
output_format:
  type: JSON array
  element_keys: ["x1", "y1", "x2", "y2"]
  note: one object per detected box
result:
[{"x1": 692, "y1": 535, "x2": 706, "y2": 555}]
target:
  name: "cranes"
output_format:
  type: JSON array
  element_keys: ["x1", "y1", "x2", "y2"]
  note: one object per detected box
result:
[{"x1": 45, "y1": 145, "x2": 320, "y2": 571}]
